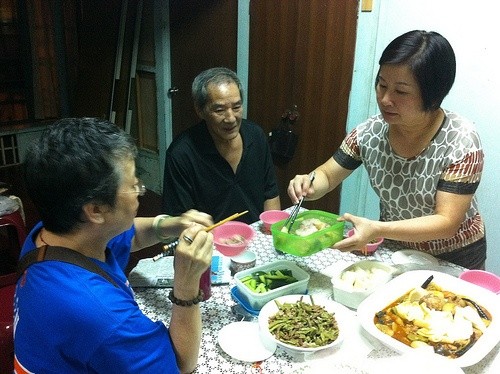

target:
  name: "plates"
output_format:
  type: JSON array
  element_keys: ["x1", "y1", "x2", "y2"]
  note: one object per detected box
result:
[
  {"x1": 258, "y1": 295, "x2": 349, "y2": 353},
  {"x1": 391, "y1": 249, "x2": 439, "y2": 266},
  {"x1": 217, "y1": 321, "x2": 278, "y2": 362},
  {"x1": 356, "y1": 270, "x2": 500, "y2": 367}
]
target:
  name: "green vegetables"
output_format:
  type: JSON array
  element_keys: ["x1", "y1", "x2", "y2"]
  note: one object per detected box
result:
[
  {"x1": 240, "y1": 269, "x2": 299, "y2": 293},
  {"x1": 268, "y1": 295, "x2": 337, "y2": 347}
]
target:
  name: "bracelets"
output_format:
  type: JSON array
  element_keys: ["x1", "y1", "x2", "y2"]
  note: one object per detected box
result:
[
  {"x1": 169, "y1": 288, "x2": 205, "y2": 306},
  {"x1": 152, "y1": 214, "x2": 175, "y2": 243}
]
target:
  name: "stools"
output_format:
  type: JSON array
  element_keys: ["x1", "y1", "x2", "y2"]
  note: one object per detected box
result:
[{"x1": 0, "y1": 210, "x2": 27, "y2": 288}]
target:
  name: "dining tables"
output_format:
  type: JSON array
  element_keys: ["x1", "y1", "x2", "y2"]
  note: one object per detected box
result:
[{"x1": 132, "y1": 205, "x2": 500, "y2": 373}]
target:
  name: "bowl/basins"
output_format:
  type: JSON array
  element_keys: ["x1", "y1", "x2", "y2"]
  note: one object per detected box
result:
[
  {"x1": 331, "y1": 259, "x2": 398, "y2": 310},
  {"x1": 347, "y1": 228, "x2": 384, "y2": 252},
  {"x1": 233, "y1": 260, "x2": 310, "y2": 310},
  {"x1": 270, "y1": 210, "x2": 346, "y2": 257},
  {"x1": 259, "y1": 210, "x2": 290, "y2": 231},
  {"x1": 210, "y1": 221, "x2": 254, "y2": 256},
  {"x1": 459, "y1": 270, "x2": 500, "y2": 295}
]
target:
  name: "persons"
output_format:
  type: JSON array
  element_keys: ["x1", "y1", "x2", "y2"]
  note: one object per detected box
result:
[
  {"x1": 157, "y1": 68, "x2": 281, "y2": 231},
  {"x1": 287, "y1": 30, "x2": 486, "y2": 272},
  {"x1": 11, "y1": 118, "x2": 214, "y2": 374}
]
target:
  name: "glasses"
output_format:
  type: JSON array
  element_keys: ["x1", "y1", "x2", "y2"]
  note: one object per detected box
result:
[{"x1": 114, "y1": 178, "x2": 148, "y2": 197}]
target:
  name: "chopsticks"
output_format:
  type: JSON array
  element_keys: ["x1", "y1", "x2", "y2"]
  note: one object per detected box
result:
[
  {"x1": 152, "y1": 210, "x2": 249, "y2": 262},
  {"x1": 282, "y1": 171, "x2": 315, "y2": 234}
]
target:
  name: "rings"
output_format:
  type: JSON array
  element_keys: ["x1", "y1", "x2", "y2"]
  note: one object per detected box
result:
[{"x1": 183, "y1": 234, "x2": 193, "y2": 243}]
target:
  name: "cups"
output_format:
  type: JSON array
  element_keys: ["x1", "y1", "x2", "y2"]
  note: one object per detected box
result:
[{"x1": 230, "y1": 251, "x2": 256, "y2": 273}]
